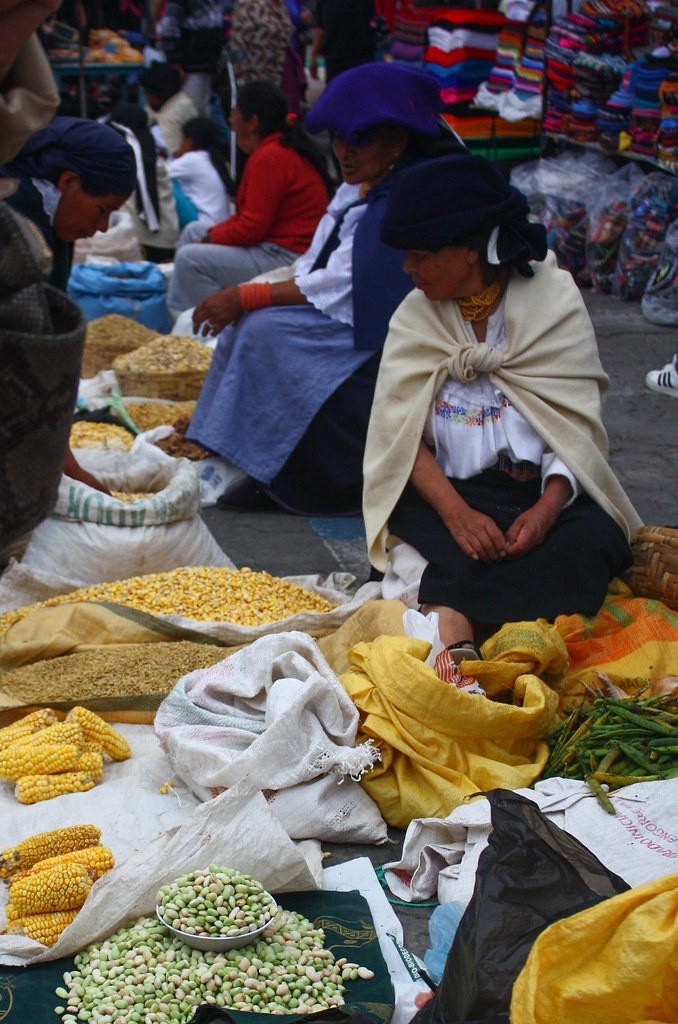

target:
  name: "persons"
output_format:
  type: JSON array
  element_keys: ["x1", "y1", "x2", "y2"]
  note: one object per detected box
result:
[
  {"x1": 139, "y1": 0, "x2": 392, "y2": 251},
  {"x1": 165, "y1": 82, "x2": 336, "y2": 311},
  {"x1": 185, "y1": 60, "x2": 475, "y2": 513},
  {"x1": 361, "y1": 153, "x2": 643, "y2": 664},
  {"x1": 0, "y1": 0, "x2": 134, "y2": 572}
]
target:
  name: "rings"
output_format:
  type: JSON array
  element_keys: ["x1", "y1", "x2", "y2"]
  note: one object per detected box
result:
[{"x1": 207, "y1": 322, "x2": 215, "y2": 329}]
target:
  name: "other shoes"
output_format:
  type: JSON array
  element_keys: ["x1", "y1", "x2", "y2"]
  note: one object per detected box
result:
[
  {"x1": 216, "y1": 487, "x2": 284, "y2": 511},
  {"x1": 445, "y1": 640, "x2": 484, "y2": 665}
]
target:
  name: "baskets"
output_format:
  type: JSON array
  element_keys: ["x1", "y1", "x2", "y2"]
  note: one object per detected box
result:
[{"x1": 623, "y1": 525, "x2": 678, "y2": 611}]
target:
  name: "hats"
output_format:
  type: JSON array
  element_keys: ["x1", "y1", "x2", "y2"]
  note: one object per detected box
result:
[
  {"x1": 16, "y1": 115, "x2": 138, "y2": 196},
  {"x1": 381, "y1": 154, "x2": 548, "y2": 279}
]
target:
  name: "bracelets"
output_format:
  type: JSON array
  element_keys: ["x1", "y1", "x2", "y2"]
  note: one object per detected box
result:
[{"x1": 240, "y1": 282, "x2": 270, "y2": 311}]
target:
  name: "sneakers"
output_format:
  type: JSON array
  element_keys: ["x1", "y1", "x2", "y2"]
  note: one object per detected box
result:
[{"x1": 646, "y1": 354, "x2": 678, "y2": 398}]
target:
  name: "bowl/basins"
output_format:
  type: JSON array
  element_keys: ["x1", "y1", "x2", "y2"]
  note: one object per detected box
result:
[{"x1": 156, "y1": 892, "x2": 278, "y2": 952}]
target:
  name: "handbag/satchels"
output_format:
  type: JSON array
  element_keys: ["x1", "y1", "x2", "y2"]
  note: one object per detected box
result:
[{"x1": 0, "y1": 202, "x2": 87, "y2": 549}]
